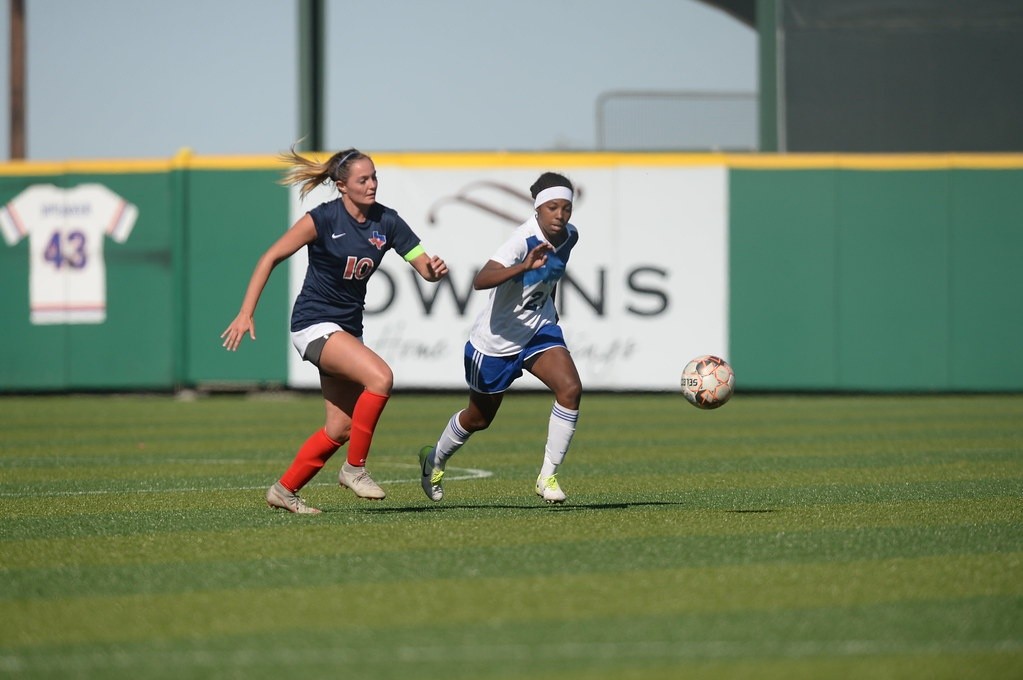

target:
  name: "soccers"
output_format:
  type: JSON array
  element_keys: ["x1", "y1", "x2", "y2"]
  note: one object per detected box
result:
[{"x1": 679, "y1": 356, "x2": 735, "y2": 411}]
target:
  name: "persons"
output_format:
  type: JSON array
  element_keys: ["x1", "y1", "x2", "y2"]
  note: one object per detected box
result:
[
  {"x1": 220, "y1": 147, "x2": 448, "y2": 513},
  {"x1": 419, "y1": 170, "x2": 582, "y2": 502}
]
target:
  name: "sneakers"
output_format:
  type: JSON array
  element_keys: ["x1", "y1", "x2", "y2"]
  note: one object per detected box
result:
[
  {"x1": 418, "y1": 445, "x2": 443, "y2": 502},
  {"x1": 266, "y1": 485, "x2": 321, "y2": 514},
  {"x1": 338, "y1": 466, "x2": 386, "y2": 500},
  {"x1": 535, "y1": 473, "x2": 566, "y2": 504}
]
